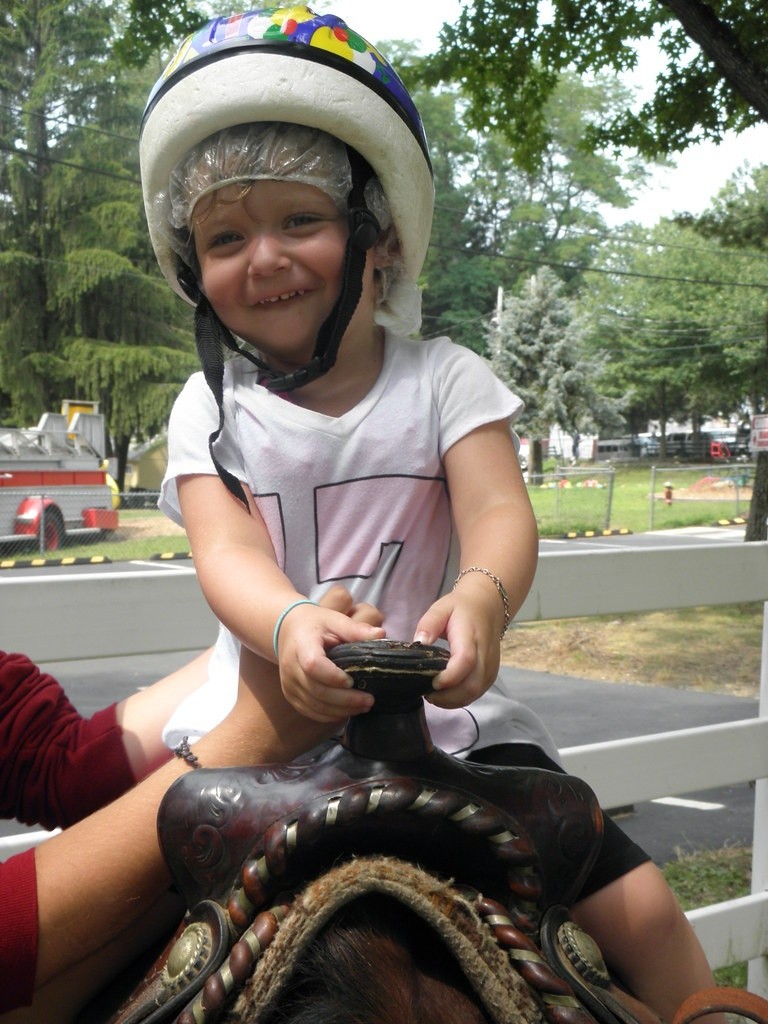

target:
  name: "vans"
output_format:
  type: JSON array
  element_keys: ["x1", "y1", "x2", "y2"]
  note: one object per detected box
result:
[
  {"x1": 665, "y1": 413, "x2": 768, "y2": 458},
  {"x1": 595, "y1": 438, "x2": 632, "y2": 461}
]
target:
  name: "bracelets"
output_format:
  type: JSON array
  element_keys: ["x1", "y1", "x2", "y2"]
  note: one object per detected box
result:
[
  {"x1": 454, "y1": 566, "x2": 509, "y2": 640},
  {"x1": 273, "y1": 599, "x2": 319, "y2": 658},
  {"x1": 175, "y1": 736, "x2": 201, "y2": 769}
]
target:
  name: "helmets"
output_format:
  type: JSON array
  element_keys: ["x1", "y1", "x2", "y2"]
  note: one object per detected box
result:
[{"x1": 138, "y1": 9, "x2": 436, "y2": 302}]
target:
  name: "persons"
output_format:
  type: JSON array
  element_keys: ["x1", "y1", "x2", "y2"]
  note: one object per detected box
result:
[
  {"x1": 0, "y1": 4, "x2": 727, "y2": 1024},
  {"x1": 0, "y1": 588, "x2": 383, "y2": 1019}
]
